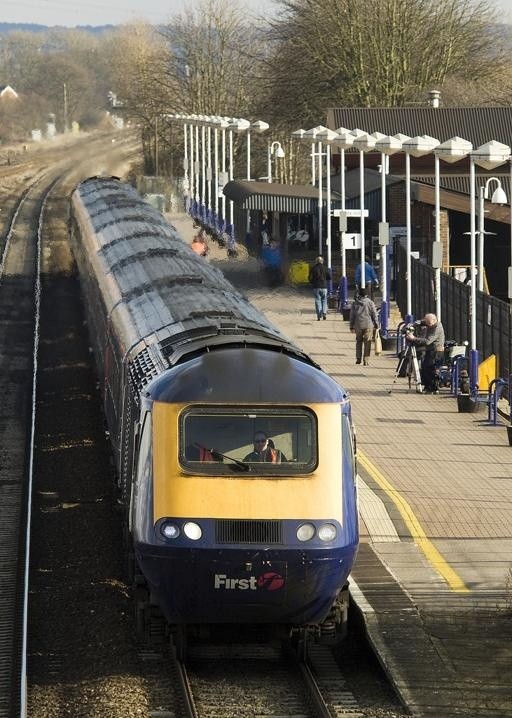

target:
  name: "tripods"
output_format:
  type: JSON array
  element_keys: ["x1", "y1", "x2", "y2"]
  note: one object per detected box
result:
[{"x1": 389, "y1": 332, "x2": 423, "y2": 394}]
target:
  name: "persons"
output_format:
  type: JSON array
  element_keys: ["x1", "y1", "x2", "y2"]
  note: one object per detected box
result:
[
  {"x1": 307, "y1": 256, "x2": 331, "y2": 320},
  {"x1": 405, "y1": 313, "x2": 445, "y2": 394},
  {"x1": 350, "y1": 289, "x2": 379, "y2": 366},
  {"x1": 242, "y1": 431, "x2": 288, "y2": 463},
  {"x1": 353, "y1": 255, "x2": 379, "y2": 303}
]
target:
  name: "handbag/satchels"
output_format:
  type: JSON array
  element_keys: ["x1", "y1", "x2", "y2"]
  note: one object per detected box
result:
[
  {"x1": 374, "y1": 329, "x2": 382, "y2": 356},
  {"x1": 354, "y1": 286, "x2": 360, "y2": 301}
]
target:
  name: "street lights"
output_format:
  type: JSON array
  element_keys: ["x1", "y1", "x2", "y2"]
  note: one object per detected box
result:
[
  {"x1": 470, "y1": 139, "x2": 510, "y2": 391},
  {"x1": 376, "y1": 133, "x2": 412, "y2": 331},
  {"x1": 478, "y1": 177, "x2": 506, "y2": 290},
  {"x1": 401, "y1": 135, "x2": 440, "y2": 324},
  {"x1": 268, "y1": 140, "x2": 284, "y2": 182},
  {"x1": 165, "y1": 113, "x2": 269, "y2": 239},
  {"x1": 293, "y1": 124, "x2": 380, "y2": 304},
  {"x1": 433, "y1": 136, "x2": 473, "y2": 317}
]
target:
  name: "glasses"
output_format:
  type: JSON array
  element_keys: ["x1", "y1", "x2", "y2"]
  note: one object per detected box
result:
[{"x1": 253, "y1": 439, "x2": 266, "y2": 443}]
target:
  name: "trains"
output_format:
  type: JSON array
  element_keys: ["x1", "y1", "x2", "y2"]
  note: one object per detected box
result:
[{"x1": 68, "y1": 175, "x2": 358, "y2": 656}]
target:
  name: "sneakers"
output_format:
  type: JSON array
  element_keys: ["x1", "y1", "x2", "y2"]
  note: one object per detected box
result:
[
  {"x1": 355, "y1": 357, "x2": 370, "y2": 366},
  {"x1": 318, "y1": 313, "x2": 326, "y2": 321}
]
